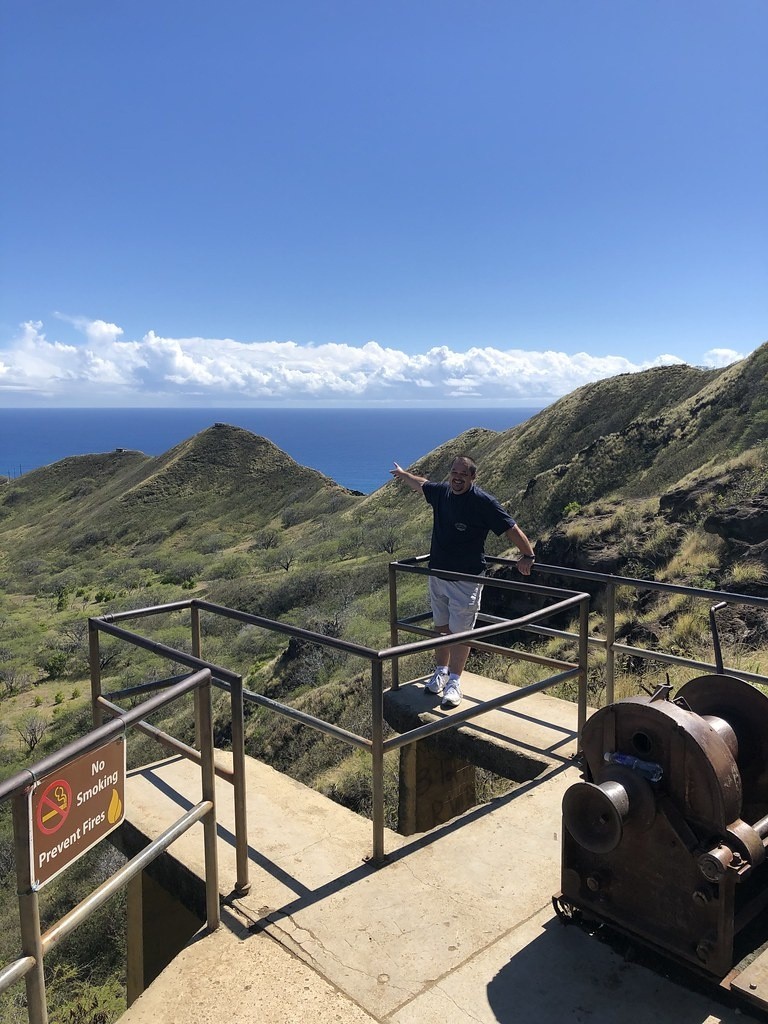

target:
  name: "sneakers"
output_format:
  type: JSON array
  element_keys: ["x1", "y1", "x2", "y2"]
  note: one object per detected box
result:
[
  {"x1": 422, "y1": 665, "x2": 449, "y2": 695},
  {"x1": 443, "y1": 678, "x2": 463, "y2": 707}
]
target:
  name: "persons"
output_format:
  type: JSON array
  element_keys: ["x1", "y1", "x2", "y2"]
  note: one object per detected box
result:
[{"x1": 388, "y1": 454, "x2": 536, "y2": 706}]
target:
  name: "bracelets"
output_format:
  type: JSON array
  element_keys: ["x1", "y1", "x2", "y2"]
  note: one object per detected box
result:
[{"x1": 523, "y1": 554, "x2": 535, "y2": 559}]
